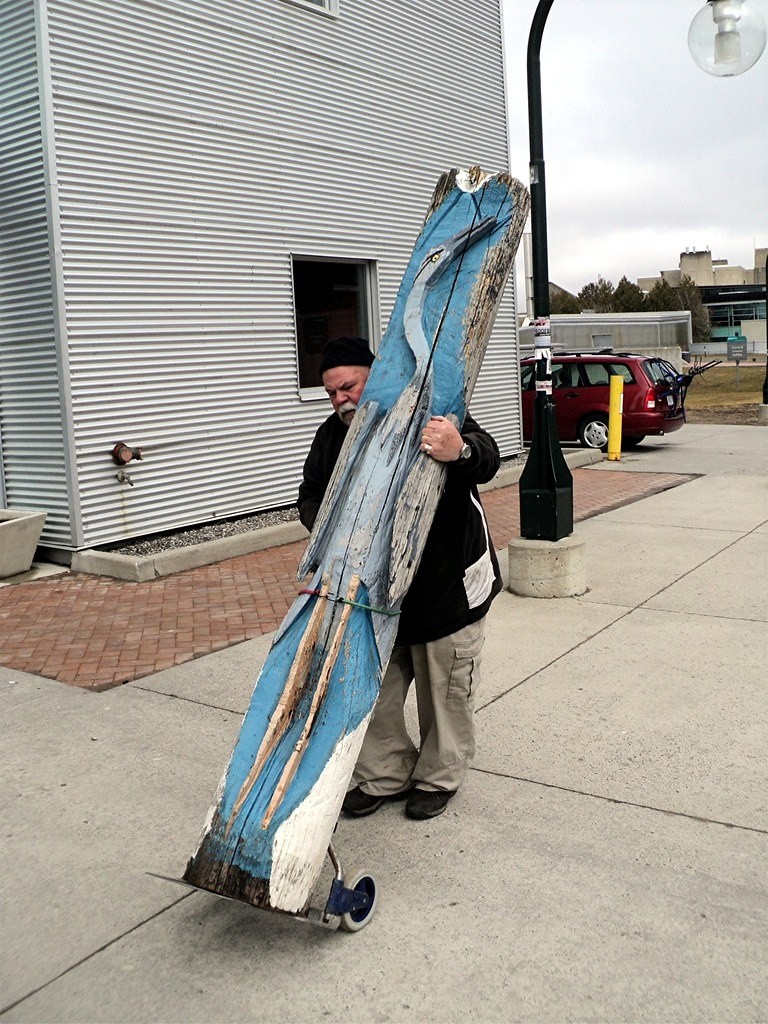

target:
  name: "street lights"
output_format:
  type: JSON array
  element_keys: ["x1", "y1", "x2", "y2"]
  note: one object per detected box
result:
[{"x1": 507, "y1": 0, "x2": 768, "y2": 600}]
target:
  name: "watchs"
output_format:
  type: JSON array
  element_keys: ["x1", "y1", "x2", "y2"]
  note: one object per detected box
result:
[{"x1": 457, "y1": 443, "x2": 472, "y2": 462}]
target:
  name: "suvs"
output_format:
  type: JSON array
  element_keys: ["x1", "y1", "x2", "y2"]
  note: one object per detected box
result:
[{"x1": 521, "y1": 348, "x2": 686, "y2": 452}]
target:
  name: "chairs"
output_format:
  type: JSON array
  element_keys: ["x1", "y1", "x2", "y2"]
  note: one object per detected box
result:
[{"x1": 557, "y1": 372, "x2": 572, "y2": 389}]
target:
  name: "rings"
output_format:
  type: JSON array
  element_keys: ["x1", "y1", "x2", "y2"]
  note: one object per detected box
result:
[{"x1": 427, "y1": 445, "x2": 433, "y2": 455}]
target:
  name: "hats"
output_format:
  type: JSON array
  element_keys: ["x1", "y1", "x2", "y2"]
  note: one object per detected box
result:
[{"x1": 319, "y1": 336, "x2": 375, "y2": 378}]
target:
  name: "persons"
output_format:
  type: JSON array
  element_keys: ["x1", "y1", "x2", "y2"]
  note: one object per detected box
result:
[{"x1": 297, "y1": 334, "x2": 499, "y2": 819}]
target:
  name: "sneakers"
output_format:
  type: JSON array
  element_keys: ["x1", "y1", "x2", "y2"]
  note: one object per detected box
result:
[
  {"x1": 405, "y1": 790, "x2": 457, "y2": 820},
  {"x1": 341, "y1": 785, "x2": 412, "y2": 817}
]
